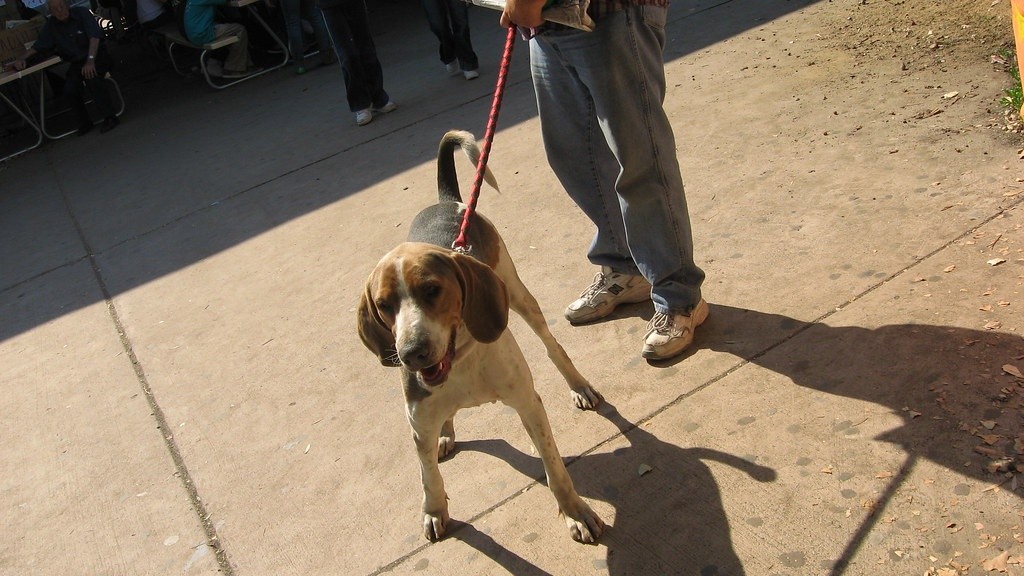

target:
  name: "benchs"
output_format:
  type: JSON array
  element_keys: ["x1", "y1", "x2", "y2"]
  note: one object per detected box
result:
[{"x1": 147, "y1": 20, "x2": 239, "y2": 90}]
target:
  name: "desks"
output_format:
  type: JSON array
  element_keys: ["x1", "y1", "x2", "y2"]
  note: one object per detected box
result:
[{"x1": 0, "y1": 56, "x2": 61, "y2": 161}]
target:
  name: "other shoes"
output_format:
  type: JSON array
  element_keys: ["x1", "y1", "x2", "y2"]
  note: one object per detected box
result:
[
  {"x1": 201, "y1": 62, "x2": 224, "y2": 76},
  {"x1": 294, "y1": 66, "x2": 305, "y2": 74},
  {"x1": 369, "y1": 102, "x2": 397, "y2": 113},
  {"x1": 304, "y1": 35, "x2": 318, "y2": 47},
  {"x1": 100, "y1": 116, "x2": 120, "y2": 134},
  {"x1": 246, "y1": 64, "x2": 265, "y2": 75},
  {"x1": 117, "y1": 37, "x2": 132, "y2": 45},
  {"x1": 356, "y1": 108, "x2": 373, "y2": 125},
  {"x1": 464, "y1": 69, "x2": 479, "y2": 80},
  {"x1": 221, "y1": 69, "x2": 253, "y2": 78},
  {"x1": 77, "y1": 120, "x2": 93, "y2": 136},
  {"x1": 446, "y1": 58, "x2": 460, "y2": 75},
  {"x1": 321, "y1": 51, "x2": 338, "y2": 65},
  {"x1": 267, "y1": 46, "x2": 285, "y2": 54}
]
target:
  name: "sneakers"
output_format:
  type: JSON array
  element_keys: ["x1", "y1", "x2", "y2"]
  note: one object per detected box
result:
[
  {"x1": 641, "y1": 298, "x2": 709, "y2": 360},
  {"x1": 565, "y1": 265, "x2": 652, "y2": 324}
]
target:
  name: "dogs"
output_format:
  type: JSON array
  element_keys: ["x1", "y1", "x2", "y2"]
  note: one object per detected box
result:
[{"x1": 356, "y1": 128, "x2": 605, "y2": 543}]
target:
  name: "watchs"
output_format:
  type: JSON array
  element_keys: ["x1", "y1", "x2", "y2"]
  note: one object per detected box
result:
[{"x1": 87, "y1": 55, "x2": 95, "y2": 60}]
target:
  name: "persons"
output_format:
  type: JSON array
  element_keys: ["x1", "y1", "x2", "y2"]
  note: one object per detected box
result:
[
  {"x1": 500, "y1": 0, "x2": 709, "y2": 361},
  {"x1": 0, "y1": 0, "x2": 480, "y2": 136}
]
